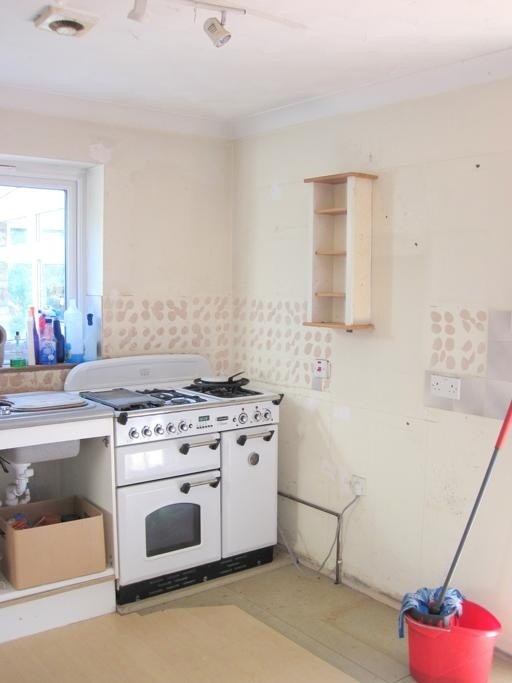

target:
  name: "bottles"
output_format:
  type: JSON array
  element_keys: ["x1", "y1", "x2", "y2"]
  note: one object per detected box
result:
[{"x1": 39, "y1": 318, "x2": 56, "y2": 366}]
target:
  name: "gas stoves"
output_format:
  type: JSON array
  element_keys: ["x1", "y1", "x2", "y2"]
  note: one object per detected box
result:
[{"x1": 115, "y1": 371, "x2": 280, "y2": 445}]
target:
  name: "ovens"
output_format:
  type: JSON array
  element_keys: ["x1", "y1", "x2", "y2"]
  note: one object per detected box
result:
[{"x1": 112, "y1": 424, "x2": 279, "y2": 606}]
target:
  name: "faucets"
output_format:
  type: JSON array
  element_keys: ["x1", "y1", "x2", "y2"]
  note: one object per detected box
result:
[{"x1": 0, "y1": 395, "x2": 16, "y2": 414}]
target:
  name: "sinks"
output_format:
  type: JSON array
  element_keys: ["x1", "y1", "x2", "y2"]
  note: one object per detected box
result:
[{"x1": 4, "y1": 392, "x2": 97, "y2": 415}]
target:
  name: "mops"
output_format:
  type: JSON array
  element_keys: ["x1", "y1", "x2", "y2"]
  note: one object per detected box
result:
[{"x1": 399, "y1": 400, "x2": 512, "y2": 640}]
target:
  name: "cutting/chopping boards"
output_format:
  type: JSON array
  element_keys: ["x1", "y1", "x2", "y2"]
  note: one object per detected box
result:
[
  {"x1": 80, "y1": 387, "x2": 161, "y2": 409},
  {"x1": 1, "y1": 391, "x2": 87, "y2": 412}
]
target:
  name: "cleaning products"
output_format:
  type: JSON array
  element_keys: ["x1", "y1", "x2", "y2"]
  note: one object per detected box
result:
[{"x1": 25, "y1": 299, "x2": 98, "y2": 363}]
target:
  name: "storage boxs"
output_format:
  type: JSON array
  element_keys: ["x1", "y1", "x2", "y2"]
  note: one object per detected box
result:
[{"x1": 0, "y1": 495, "x2": 107, "y2": 591}]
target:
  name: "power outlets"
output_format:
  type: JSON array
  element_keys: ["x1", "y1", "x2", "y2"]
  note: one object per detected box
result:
[
  {"x1": 430, "y1": 375, "x2": 444, "y2": 398},
  {"x1": 444, "y1": 378, "x2": 460, "y2": 399}
]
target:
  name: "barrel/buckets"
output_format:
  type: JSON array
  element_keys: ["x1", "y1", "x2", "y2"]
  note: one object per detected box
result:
[{"x1": 404, "y1": 598, "x2": 504, "y2": 682}]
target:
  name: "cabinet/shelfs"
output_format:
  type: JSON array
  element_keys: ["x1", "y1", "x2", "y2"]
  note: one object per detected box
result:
[{"x1": 302, "y1": 171, "x2": 378, "y2": 333}]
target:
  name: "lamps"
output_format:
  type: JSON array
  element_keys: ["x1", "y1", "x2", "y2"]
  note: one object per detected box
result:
[{"x1": 202, "y1": 10, "x2": 231, "y2": 49}]
